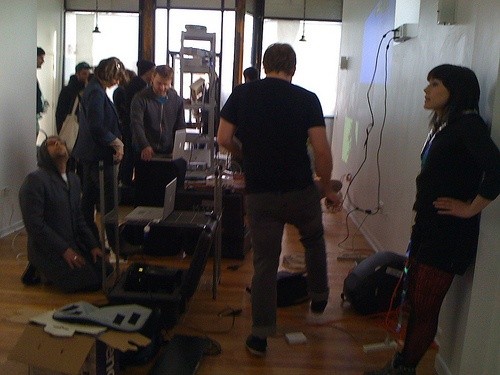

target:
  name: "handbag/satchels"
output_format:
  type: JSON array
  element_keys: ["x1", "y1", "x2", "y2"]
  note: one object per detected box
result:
[{"x1": 57, "y1": 94, "x2": 81, "y2": 155}]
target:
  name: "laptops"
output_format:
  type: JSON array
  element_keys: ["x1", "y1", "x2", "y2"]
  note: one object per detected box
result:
[
  {"x1": 124, "y1": 177, "x2": 178, "y2": 223},
  {"x1": 151, "y1": 129, "x2": 192, "y2": 162}
]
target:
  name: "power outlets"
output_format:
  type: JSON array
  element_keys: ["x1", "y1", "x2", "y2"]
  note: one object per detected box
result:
[{"x1": 378, "y1": 201, "x2": 385, "y2": 214}]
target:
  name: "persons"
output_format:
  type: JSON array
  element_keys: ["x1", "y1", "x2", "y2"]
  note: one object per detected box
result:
[
  {"x1": 54, "y1": 56, "x2": 157, "y2": 254},
  {"x1": 243, "y1": 67, "x2": 260, "y2": 82},
  {"x1": 128, "y1": 66, "x2": 185, "y2": 159},
  {"x1": 18, "y1": 135, "x2": 114, "y2": 292},
  {"x1": 363, "y1": 64, "x2": 500, "y2": 375},
  {"x1": 215, "y1": 43, "x2": 333, "y2": 356},
  {"x1": 36, "y1": 48, "x2": 45, "y2": 137}
]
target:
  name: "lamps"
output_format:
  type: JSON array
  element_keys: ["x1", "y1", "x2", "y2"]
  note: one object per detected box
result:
[
  {"x1": 299, "y1": 0, "x2": 306, "y2": 41},
  {"x1": 93, "y1": 0, "x2": 101, "y2": 33}
]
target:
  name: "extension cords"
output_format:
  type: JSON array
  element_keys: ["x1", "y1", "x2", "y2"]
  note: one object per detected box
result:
[{"x1": 362, "y1": 341, "x2": 399, "y2": 353}]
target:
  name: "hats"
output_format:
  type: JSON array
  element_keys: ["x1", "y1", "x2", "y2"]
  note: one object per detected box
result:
[{"x1": 75, "y1": 62, "x2": 93, "y2": 71}]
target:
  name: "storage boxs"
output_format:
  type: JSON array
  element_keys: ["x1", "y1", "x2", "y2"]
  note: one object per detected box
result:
[{"x1": 6, "y1": 306, "x2": 150, "y2": 375}]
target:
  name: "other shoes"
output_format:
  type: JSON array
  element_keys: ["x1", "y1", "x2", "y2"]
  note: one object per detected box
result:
[
  {"x1": 244, "y1": 334, "x2": 268, "y2": 356},
  {"x1": 113, "y1": 242, "x2": 144, "y2": 256},
  {"x1": 311, "y1": 300, "x2": 327, "y2": 313},
  {"x1": 363, "y1": 350, "x2": 418, "y2": 375},
  {"x1": 20, "y1": 262, "x2": 39, "y2": 284}
]
